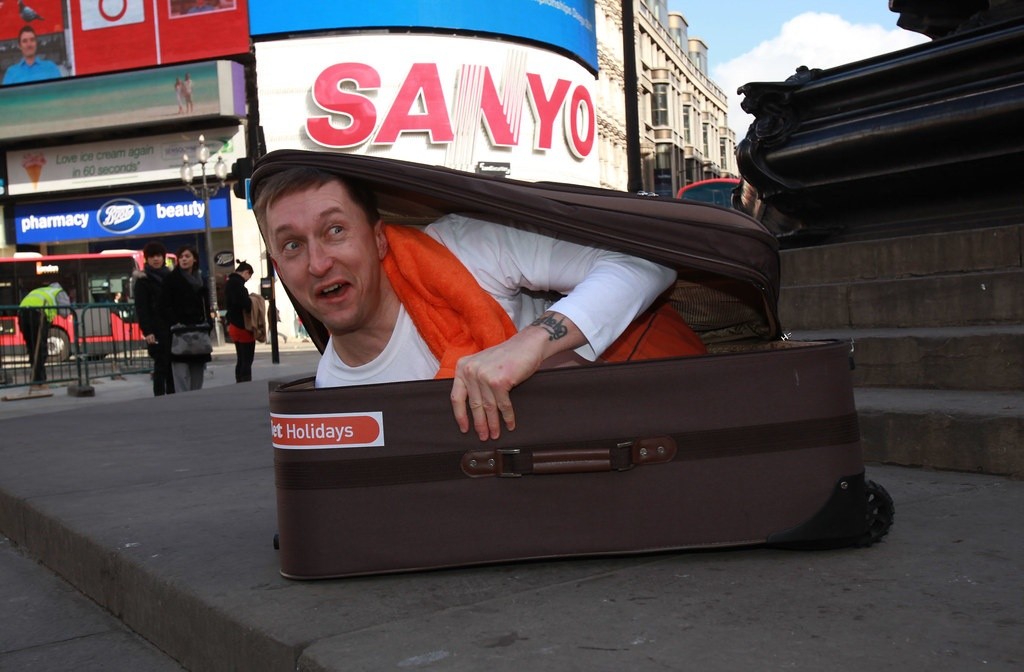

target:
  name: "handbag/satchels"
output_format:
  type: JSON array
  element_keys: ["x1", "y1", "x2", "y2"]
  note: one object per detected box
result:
[
  {"x1": 219, "y1": 316, "x2": 262, "y2": 343},
  {"x1": 169, "y1": 321, "x2": 213, "y2": 358}
]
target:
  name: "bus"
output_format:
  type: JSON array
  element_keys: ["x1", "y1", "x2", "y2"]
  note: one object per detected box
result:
[
  {"x1": 0, "y1": 249, "x2": 177, "y2": 363},
  {"x1": 676, "y1": 178, "x2": 743, "y2": 213}
]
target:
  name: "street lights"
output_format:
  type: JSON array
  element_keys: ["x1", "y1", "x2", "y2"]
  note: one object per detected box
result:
[{"x1": 178, "y1": 134, "x2": 228, "y2": 346}]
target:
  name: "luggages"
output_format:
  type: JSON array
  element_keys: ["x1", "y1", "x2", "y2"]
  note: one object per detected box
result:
[{"x1": 248, "y1": 148, "x2": 896, "y2": 580}]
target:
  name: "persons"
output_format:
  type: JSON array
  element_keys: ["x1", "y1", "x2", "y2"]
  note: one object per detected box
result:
[
  {"x1": 223, "y1": 259, "x2": 257, "y2": 384},
  {"x1": 252, "y1": 159, "x2": 708, "y2": 441},
  {"x1": 3, "y1": 25, "x2": 65, "y2": 85},
  {"x1": 133, "y1": 242, "x2": 215, "y2": 391},
  {"x1": 18, "y1": 278, "x2": 77, "y2": 389},
  {"x1": 174, "y1": 70, "x2": 194, "y2": 116}
]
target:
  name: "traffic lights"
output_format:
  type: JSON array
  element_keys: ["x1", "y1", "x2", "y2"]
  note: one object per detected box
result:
[{"x1": 232, "y1": 157, "x2": 253, "y2": 200}]
